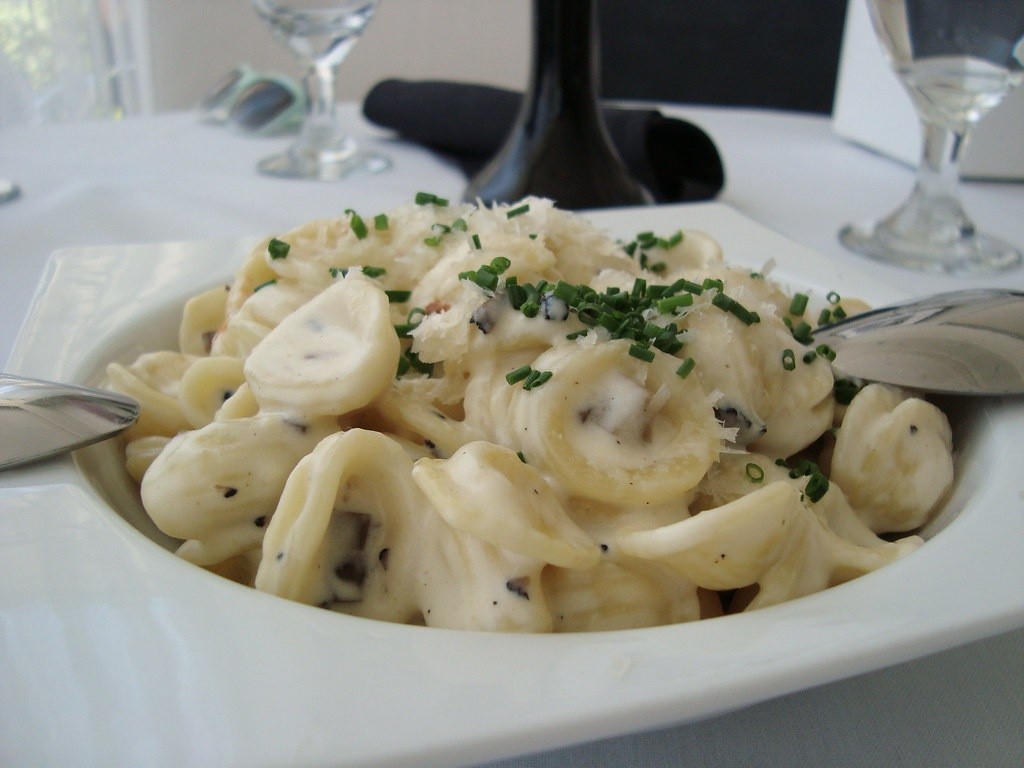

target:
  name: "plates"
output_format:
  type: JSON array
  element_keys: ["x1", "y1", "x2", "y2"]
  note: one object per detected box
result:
[{"x1": 0, "y1": 199, "x2": 1024, "y2": 768}]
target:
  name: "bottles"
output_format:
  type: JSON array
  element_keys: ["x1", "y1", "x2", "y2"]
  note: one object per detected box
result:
[{"x1": 461, "y1": 0, "x2": 655, "y2": 211}]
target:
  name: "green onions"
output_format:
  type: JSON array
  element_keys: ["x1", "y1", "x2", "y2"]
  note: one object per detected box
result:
[{"x1": 255, "y1": 191, "x2": 860, "y2": 506}]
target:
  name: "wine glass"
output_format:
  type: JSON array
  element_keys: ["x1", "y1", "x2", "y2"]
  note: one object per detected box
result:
[
  {"x1": 836, "y1": 0, "x2": 1024, "y2": 274},
  {"x1": 251, "y1": 0, "x2": 393, "y2": 186}
]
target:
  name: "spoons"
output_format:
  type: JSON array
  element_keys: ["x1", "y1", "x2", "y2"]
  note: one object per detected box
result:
[
  {"x1": 0, "y1": 373, "x2": 142, "y2": 471},
  {"x1": 809, "y1": 286, "x2": 1024, "y2": 397}
]
